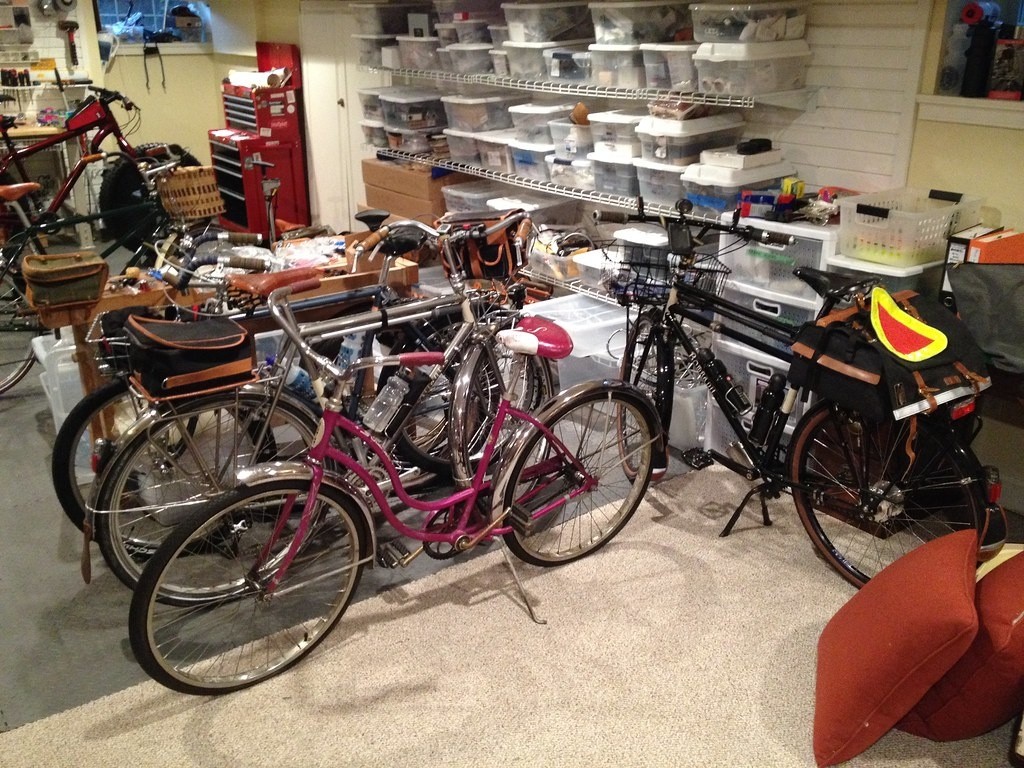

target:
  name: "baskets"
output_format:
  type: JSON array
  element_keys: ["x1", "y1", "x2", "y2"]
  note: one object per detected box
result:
[
  {"x1": 833, "y1": 187, "x2": 985, "y2": 263},
  {"x1": 156, "y1": 161, "x2": 226, "y2": 220},
  {"x1": 601, "y1": 245, "x2": 731, "y2": 307},
  {"x1": 86, "y1": 304, "x2": 185, "y2": 374}
]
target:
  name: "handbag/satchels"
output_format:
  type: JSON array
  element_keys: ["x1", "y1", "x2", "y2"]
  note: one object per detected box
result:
[
  {"x1": 23, "y1": 249, "x2": 110, "y2": 309},
  {"x1": 789, "y1": 289, "x2": 996, "y2": 427},
  {"x1": 434, "y1": 208, "x2": 528, "y2": 278},
  {"x1": 121, "y1": 314, "x2": 258, "y2": 396}
]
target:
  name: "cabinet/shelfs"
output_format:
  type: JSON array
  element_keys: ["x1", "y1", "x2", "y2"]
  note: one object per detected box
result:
[{"x1": 358, "y1": 64, "x2": 818, "y2": 338}]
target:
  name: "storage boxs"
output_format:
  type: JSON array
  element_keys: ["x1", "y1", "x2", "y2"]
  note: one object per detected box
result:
[
  {"x1": 31, "y1": 333, "x2": 91, "y2": 468},
  {"x1": 351, "y1": 0, "x2": 947, "y2": 470}
]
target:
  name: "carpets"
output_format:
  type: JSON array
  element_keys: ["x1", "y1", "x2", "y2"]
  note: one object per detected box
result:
[{"x1": 0, "y1": 456, "x2": 1024, "y2": 768}]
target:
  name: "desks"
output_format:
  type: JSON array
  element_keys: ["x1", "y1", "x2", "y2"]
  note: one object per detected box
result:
[
  {"x1": 0, "y1": 124, "x2": 96, "y2": 252},
  {"x1": 37, "y1": 257, "x2": 420, "y2": 450},
  {"x1": 810, "y1": 362, "x2": 1024, "y2": 539}
]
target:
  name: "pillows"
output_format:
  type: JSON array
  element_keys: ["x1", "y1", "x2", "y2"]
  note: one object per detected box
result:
[
  {"x1": 893, "y1": 551, "x2": 1024, "y2": 742},
  {"x1": 812, "y1": 529, "x2": 978, "y2": 766}
]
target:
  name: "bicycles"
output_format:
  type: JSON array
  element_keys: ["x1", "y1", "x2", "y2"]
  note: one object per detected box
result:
[
  {"x1": 0, "y1": 143, "x2": 246, "y2": 395},
  {"x1": 592, "y1": 197, "x2": 1007, "y2": 591},
  {"x1": 51, "y1": 210, "x2": 664, "y2": 696},
  {"x1": 0, "y1": 85, "x2": 214, "y2": 256}
]
